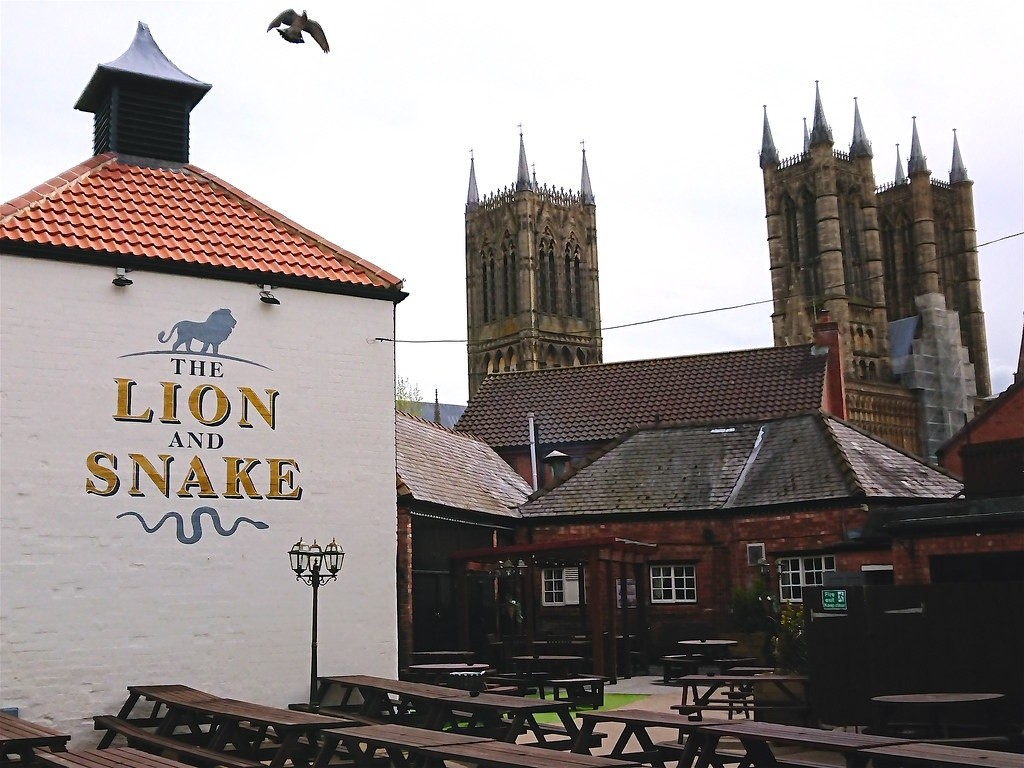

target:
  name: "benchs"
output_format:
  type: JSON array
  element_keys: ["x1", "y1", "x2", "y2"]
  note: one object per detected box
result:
[
  {"x1": 389, "y1": 697, "x2": 608, "y2": 747},
  {"x1": 208, "y1": 714, "x2": 390, "y2": 757},
  {"x1": 914, "y1": 735, "x2": 1008, "y2": 750},
  {"x1": 94, "y1": 714, "x2": 268, "y2": 767},
  {"x1": 654, "y1": 742, "x2": 846, "y2": 767},
  {"x1": 288, "y1": 702, "x2": 386, "y2": 725},
  {"x1": 399, "y1": 631, "x2": 807, "y2": 722}
]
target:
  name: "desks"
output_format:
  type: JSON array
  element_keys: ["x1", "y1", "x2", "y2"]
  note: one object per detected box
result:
[
  {"x1": 678, "y1": 674, "x2": 808, "y2": 744},
  {"x1": 313, "y1": 722, "x2": 495, "y2": 767},
  {"x1": 410, "y1": 650, "x2": 476, "y2": 663},
  {"x1": 573, "y1": 634, "x2": 637, "y2": 675},
  {"x1": 409, "y1": 663, "x2": 489, "y2": 691},
  {"x1": 695, "y1": 719, "x2": 919, "y2": 767},
  {"x1": 420, "y1": 741, "x2": 642, "y2": 767},
  {"x1": 858, "y1": 741, "x2": 1024, "y2": 767},
  {"x1": 729, "y1": 666, "x2": 775, "y2": 720},
  {"x1": 510, "y1": 654, "x2": 584, "y2": 672},
  {"x1": 533, "y1": 640, "x2": 591, "y2": 655},
  {"x1": 870, "y1": 690, "x2": 1005, "y2": 738},
  {"x1": 318, "y1": 673, "x2": 592, "y2": 755},
  {"x1": 570, "y1": 709, "x2": 753, "y2": 767},
  {"x1": 677, "y1": 639, "x2": 739, "y2": 673},
  {"x1": 35, "y1": 746, "x2": 199, "y2": 767},
  {"x1": 128, "y1": 683, "x2": 363, "y2": 767},
  {"x1": 0, "y1": 709, "x2": 72, "y2": 754}
]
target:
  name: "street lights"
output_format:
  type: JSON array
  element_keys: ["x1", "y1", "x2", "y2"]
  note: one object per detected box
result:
[{"x1": 288, "y1": 537, "x2": 345, "y2": 706}]
